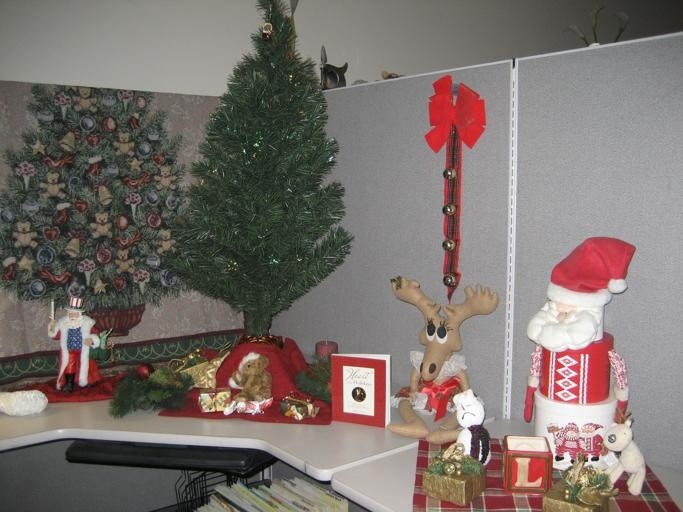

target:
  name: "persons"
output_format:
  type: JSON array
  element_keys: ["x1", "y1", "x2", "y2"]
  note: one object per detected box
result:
[
  {"x1": 48, "y1": 296, "x2": 99, "y2": 393},
  {"x1": 524, "y1": 237, "x2": 636, "y2": 471}
]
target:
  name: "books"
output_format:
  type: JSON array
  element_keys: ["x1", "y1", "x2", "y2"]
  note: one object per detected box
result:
[
  {"x1": 192, "y1": 478, "x2": 350, "y2": 512},
  {"x1": 330, "y1": 353, "x2": 392, "y2": 427}
]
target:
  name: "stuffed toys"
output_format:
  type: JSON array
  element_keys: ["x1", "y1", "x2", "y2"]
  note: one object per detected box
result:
[
  {"x1": 601, "y1": 414, "x2": 645, "y2": 495},
  {"x1": 439, "y1": 392, "x2": 490, "y2": 468},
  {"x1": 387, "y1": 276, "x2": 500, "y2": 444},
  {"x1": 233, "y1": 353, "x2": 270, "y2": 407}
]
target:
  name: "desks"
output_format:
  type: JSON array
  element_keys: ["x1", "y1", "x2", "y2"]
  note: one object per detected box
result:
[
  {"x1": 0, "y1": 364, "x2": 454, "y2": 512},
  {"x1": 332, "y1": 420, "x2": 677, "y2": 512}
]
